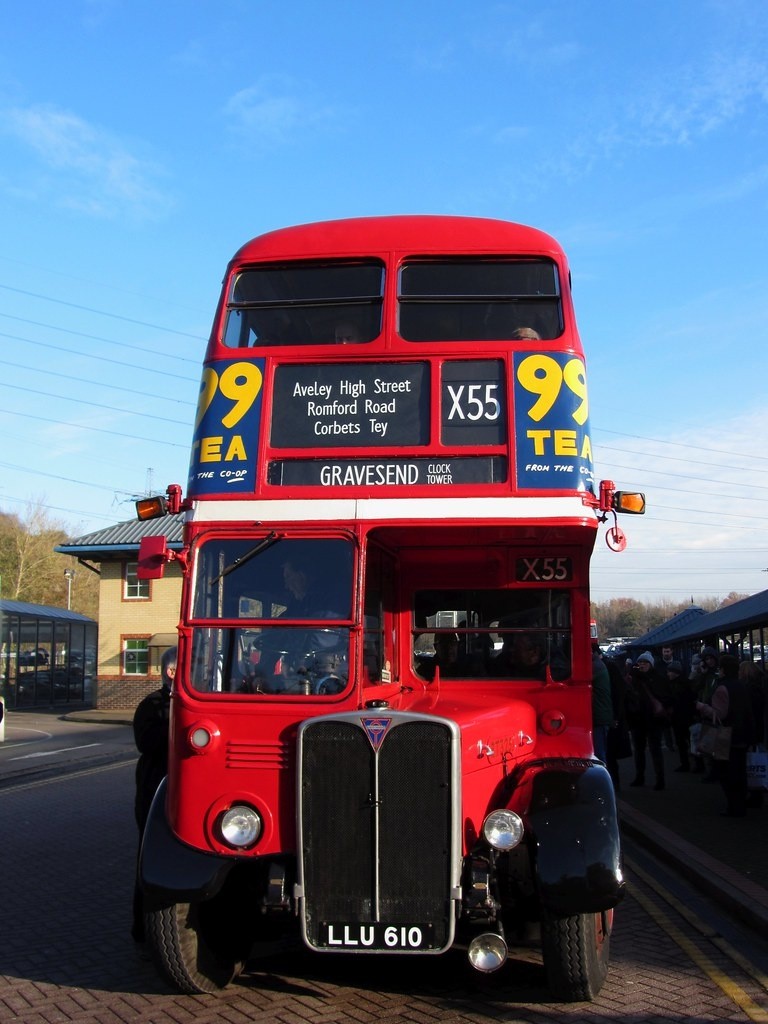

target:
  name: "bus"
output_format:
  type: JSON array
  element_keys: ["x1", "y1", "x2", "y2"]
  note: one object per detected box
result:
[{"x1": 137, "y1": 214, "x2": 646, "y2": 1002}]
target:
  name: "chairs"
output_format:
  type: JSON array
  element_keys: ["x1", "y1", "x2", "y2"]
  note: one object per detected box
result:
[{"x1": 244, "y1": 608, "x2": 379, "y2": 702}]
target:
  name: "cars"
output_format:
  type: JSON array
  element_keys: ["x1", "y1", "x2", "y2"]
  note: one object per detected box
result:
[{"x1": 597, "y1": 634, "x2": 768, "y2": 664}]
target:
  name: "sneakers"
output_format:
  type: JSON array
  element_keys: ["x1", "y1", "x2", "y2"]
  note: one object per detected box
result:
[
  {"x1": 630, "y1": 776, "x2": 646, "y2": 787},
  {"x1": 699, "y1": 774, "x2": 718, "y2": 784},
  {"x1": 746, "y1": 796, "x2": 764, "y2": 807},
  {"x1": 674, "y1": 764, "x2": 690, "y2": 772},
  {"x1": 720, "y1": 806, "x2": 746, "y2": 817},
  {"x1": 653, "y1": 777, "x2": 665, "y2": 791}
]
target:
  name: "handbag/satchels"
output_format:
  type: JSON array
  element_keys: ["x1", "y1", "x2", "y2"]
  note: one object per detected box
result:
[
  {"x1": 695, "y1": 712, "x2": 733, "y2": 762},
  {"x1": 651, "y1": 697, "x2": 668, "y2": 717},
  {"x1": 746, "y1": 746, "x2": 768, "y2": 791}
]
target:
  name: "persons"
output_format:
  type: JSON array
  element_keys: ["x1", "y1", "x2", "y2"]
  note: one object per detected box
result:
[
  {"x1": 252, "y1": 557, "x2": 346, "y2": 677},
  {"x1": 417, "y1": 620, "x2": 768, "y2": 817},
  {"x1": 252, "y1": 318, "x2": 543, "y2": 348},
  {"x1": 132, "y1": 642, "x2": 180, "y2": 964}
]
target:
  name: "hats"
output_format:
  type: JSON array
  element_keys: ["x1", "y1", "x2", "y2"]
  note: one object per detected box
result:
[
  {"x1": 636, "y1": 651, "x2": 654, "y2": 668},
  {"x1": 667, "y1": 661, "x2": 683, "y2": 671}
]
